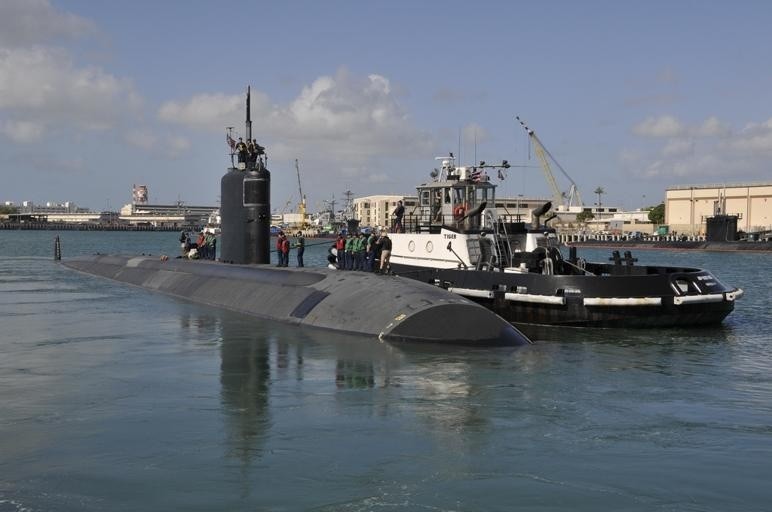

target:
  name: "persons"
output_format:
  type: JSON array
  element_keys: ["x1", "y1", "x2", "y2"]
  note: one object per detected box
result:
[
  {"x1": 334, "y1": 229, "x2": 392, "y2": 276},
  {"x1": 231, "y1": 136, "x2": 264, "y2": 171},
  {"x1": 276, "y1": 231, "x2": 285, "y2": 267},
  {"x1": 178, "y1": 230, "x2": 217, "y2": 260},
  {"x1": 292, "y1": 231, "x2": 306, "y2": 267},
  {"x1": 281, "y1": 235, "x2": 290, "y2": 267},
  {"x1": 392, "y1": 201, "x2": 404, "y2": 233},
  {"x1": 454, "y1": 202, "x2": 468, "y2": 231}
]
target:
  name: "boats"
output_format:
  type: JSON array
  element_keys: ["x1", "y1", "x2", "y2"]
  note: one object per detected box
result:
[{"x1": 327, "y1": 151, "x2": 745, "y2": 331}]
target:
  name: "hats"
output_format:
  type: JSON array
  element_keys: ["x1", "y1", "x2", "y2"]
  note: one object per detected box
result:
[
  {"x1": 381, "y1": 232, "x2": 387, "y2": 237},
  {"x1": 278, "y1": 231, "x2": 302, "y2": 238},
  {"x1": 337, "y1": 231, "x2": 376, "y2": 236},
  {"x1": 198, "y1": 231, "x2": 215, "y2": 236}
]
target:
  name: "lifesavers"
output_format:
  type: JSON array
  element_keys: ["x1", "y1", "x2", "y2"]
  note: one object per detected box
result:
[{"x1": 454, "y1": 207, "x2": 465, "y2": 220}]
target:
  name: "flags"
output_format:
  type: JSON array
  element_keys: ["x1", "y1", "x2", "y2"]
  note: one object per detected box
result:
[{"x1": 472, "y1": 171, "x2": 481, "y2": 182}]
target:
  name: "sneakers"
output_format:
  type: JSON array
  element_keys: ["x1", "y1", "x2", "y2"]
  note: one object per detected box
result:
[
  {"x1": 296, "y1": 264, "x2": 305, "y2": 267},
  {"x1": 336, "y1": 267, "x2": 367, "y2": 272},
  {"x1": 200, "y1": 257, "x2": 215, "y2": 261},
  {"x1": 275, "y1": 264, "x2": 288, "y2": 267}
]
